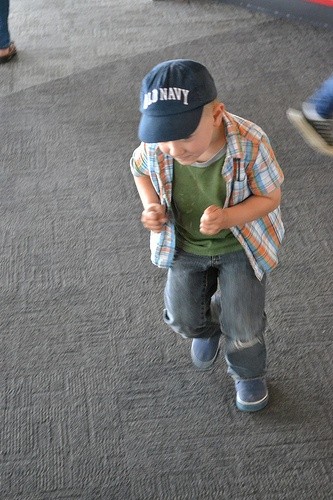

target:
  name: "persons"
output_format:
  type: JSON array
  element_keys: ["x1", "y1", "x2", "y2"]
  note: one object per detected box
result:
[
  {"x1": 0, "y1": 0, "x2": 16, "y2": 64},
  {"x1": 287, "y1": 73, "x2": 333, "y2": 155},
  {"x1": 127, "y1": 60, "x2": 286, "y2": 413}
]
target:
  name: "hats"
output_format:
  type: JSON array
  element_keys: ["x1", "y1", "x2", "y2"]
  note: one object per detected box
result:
[{"x1": 136, "y1": 59, "x2": 218, "y2": 145}]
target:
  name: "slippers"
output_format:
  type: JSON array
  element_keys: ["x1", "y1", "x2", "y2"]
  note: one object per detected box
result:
[{"x1": 285, "y1": 102, "x2": 333, "y2": 157}]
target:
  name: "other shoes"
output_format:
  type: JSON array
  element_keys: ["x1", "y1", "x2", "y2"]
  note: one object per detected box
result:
[
  {"x1": 190, "y1": 339, "x2": 222, "y2": 368},
  {"x1": 0, "y1": 42, "x2": 18, "y2": 64},
  {"x1": 232, "y1": 377, "x2": 270, "y2": 411}
]
